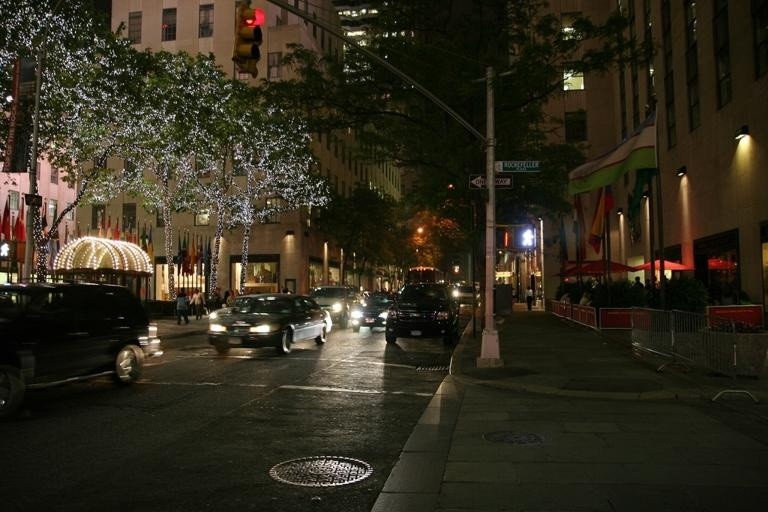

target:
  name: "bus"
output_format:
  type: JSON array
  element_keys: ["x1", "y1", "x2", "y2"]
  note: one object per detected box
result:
[{"x1": 407, "y1": 266, "x2": 446, "y2": 284}]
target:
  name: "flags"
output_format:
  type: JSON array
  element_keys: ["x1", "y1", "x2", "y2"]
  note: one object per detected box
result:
[
  {"x1": 587, "y1": 185, "x2": 615, "y2": 255},
  {"x1": 571, "y1": 195, "x2": 587, "y2": 236},
  {"x1": 624, "y1": 168, "x2": 657, "y2": 222}
]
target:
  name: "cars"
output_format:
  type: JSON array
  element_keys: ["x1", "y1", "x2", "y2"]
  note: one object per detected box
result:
[
  {"x1": 0, "y1": 281, "x2": 166, "y2": 405},
  {"x1": 204, "y1": 282, "x2": 483, "y2": 356}
]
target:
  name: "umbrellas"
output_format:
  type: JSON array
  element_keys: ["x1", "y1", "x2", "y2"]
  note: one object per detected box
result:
[
  {"x1": 551, "y1": 266, "x2": 584, "y2": 282},
  {"x1": 570, "y1": 258, "x2": 642, "y2": 286},
  {"x1": 626, "y1": 256, "x2": 696, "y2": 272}
]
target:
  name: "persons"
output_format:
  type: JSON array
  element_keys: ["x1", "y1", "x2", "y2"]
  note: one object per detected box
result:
[
  {"x1": 175, "y1": 287, "x2": 191, "y2": 325},
  {"x1": 210, "y1": 285, "x2": 238, "y2": 311},
  {"x1": 189, "y1": 286, "x2": 206, "y2": 321},
  {"x1": 523, "y1": 285, "x2": 533, "y2": 311},
  {"x1": 511, "y1": 288, "x2": 517, "y2": 303}
]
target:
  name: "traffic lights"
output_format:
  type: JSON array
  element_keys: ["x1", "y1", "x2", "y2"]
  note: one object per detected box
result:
[
  {"x1": 417, "y1": 221, "x2": 425, "y2": 238},
  {"x1": 0, "y1": 240, "x2": 15, "y2": 260},
  {"x1": 503, "y1": 232, "x2": 509, "y2": 248},
  {"x1": 232, "y1": 1, "x2": 265, "y2": 78},
  {"x1": 513, "y1": 224, "x2": 538, "y2": 249}
]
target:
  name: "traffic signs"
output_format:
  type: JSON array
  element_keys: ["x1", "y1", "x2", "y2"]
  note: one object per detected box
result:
[
  {"x1": 495, "y1": 161, "x2": 540, "y2": 174},
  {"x1": 468, "y1": 173, "x2": 513, "y2": 189}
]
